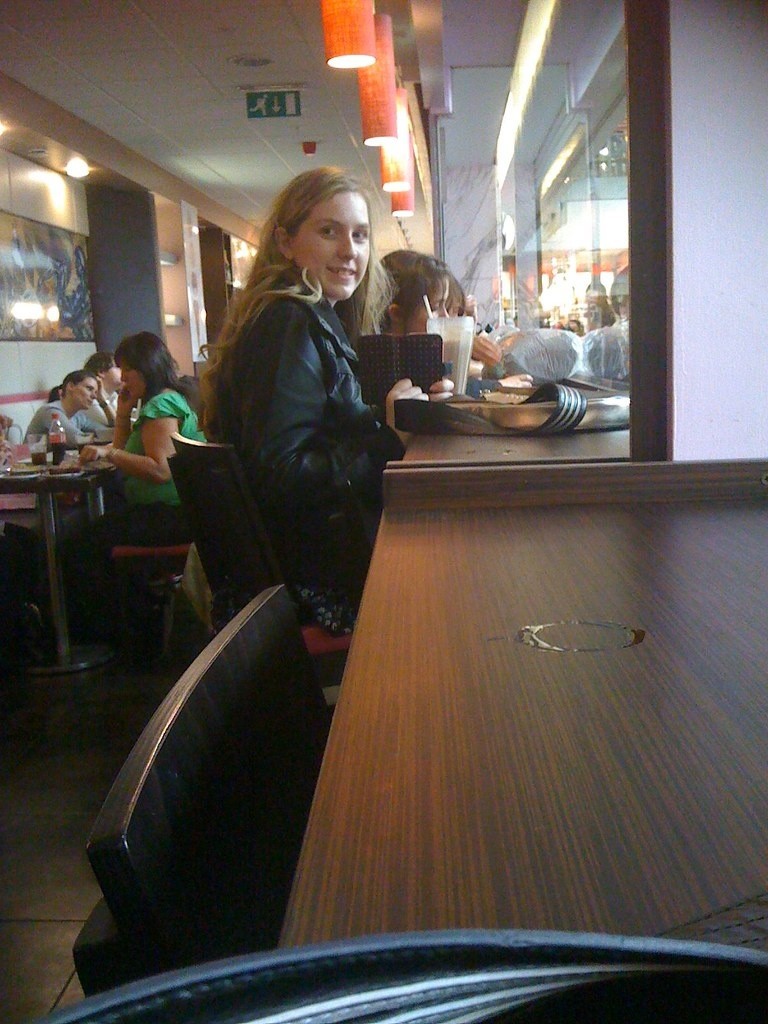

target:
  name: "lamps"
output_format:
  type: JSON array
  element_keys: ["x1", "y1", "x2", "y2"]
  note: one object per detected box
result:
[{"x1": 319, "y1": 0, "x2": 419, "y2": 219}]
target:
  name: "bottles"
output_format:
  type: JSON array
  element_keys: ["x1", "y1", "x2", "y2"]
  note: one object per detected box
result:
[{"x1": 47, "y1": 413, "x2": 66, "y2": 466}]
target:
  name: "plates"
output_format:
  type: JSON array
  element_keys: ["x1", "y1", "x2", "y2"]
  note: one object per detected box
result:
[{"x1": 0, "y1": 473, "x2": 83, "y2": 482}]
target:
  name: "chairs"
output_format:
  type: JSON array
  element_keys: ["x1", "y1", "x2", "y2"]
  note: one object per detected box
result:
[
  {"x1": 72, "y1": 584, "x2": 330, "y2": 999},
  {"x1": 168, "y1": 428, "x2": 356, "y2": 709}
]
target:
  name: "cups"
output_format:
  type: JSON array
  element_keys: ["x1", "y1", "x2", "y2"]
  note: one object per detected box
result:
[
  {"x1": 26, "y1": 433, "x2": 48, "y2": 465},
  {"x1": 425, "y1": 316, "x2": 474, "y2": 394}
]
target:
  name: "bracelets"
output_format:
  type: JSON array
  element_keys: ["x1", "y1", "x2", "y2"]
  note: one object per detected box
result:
[
  {"x1": 105, "y1": 448, "x2": 117, "y2": 458},
  {"x1": 100, "y1": 402, "x2": 108, "y2": 409}
]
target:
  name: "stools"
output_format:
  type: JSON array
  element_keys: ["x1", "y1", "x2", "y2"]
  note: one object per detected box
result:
[{"x1": 111, "y1": 545, "x2": 196, "y2": 673}]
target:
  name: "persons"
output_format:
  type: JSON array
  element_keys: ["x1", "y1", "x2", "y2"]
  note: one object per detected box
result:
[
  {"x1": 197, "y1": 167, "x2": 533, "y2": 641},
  {"x1": 0, "y1": 330, "x2": 207, "y2": 646}
]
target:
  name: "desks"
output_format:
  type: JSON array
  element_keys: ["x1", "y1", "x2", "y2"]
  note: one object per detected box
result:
[
  {"x1": 284, "y1": 384, "x2": 767, "y2": 959},
  {"x1": 0, "y1": 442, "x2": 120, "y2": 674}
]
